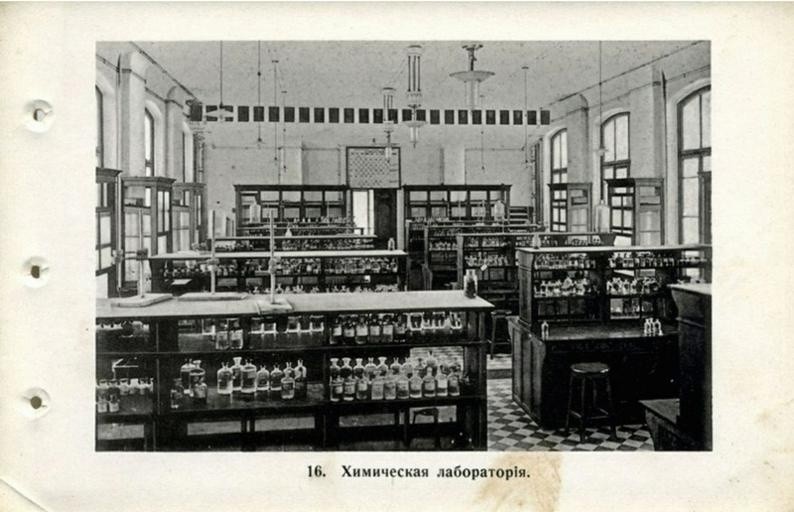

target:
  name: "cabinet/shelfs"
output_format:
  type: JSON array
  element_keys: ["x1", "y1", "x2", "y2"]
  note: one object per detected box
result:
[{"x1": 96, "y1": 219, "x2": 712, "y2": 450}]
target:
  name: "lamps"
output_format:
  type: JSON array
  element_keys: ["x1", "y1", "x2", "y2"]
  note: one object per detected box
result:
[{"x1": 211, "y1": 41, "x2": 608, "y2": 174}]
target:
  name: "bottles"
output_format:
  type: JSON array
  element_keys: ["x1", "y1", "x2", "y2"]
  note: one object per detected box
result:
[
  {"x1": 643, "y1": 317, "x2": 661, "y2": 336},
  {"x1": 96, "y1": 311, "x2": 463, "y2": 415},
  {"x1": 172, "y1": 257, "x2": 398, "y2": 274},
  {"x1": 540, "y1": 319, "x2": 548, "y2": 332},
  {"x1": 494, "y1": 200, "x2": 505, "y2": 221},
  {"x1": 463, "y1": 269, "x2": 477, "y2": 298},
  {"x1": 535, "y1": 252, "x2": 675, "y2": 298},
  {"x1": 388, "y1": 237, "x2": 396, "y2": 251}
]
